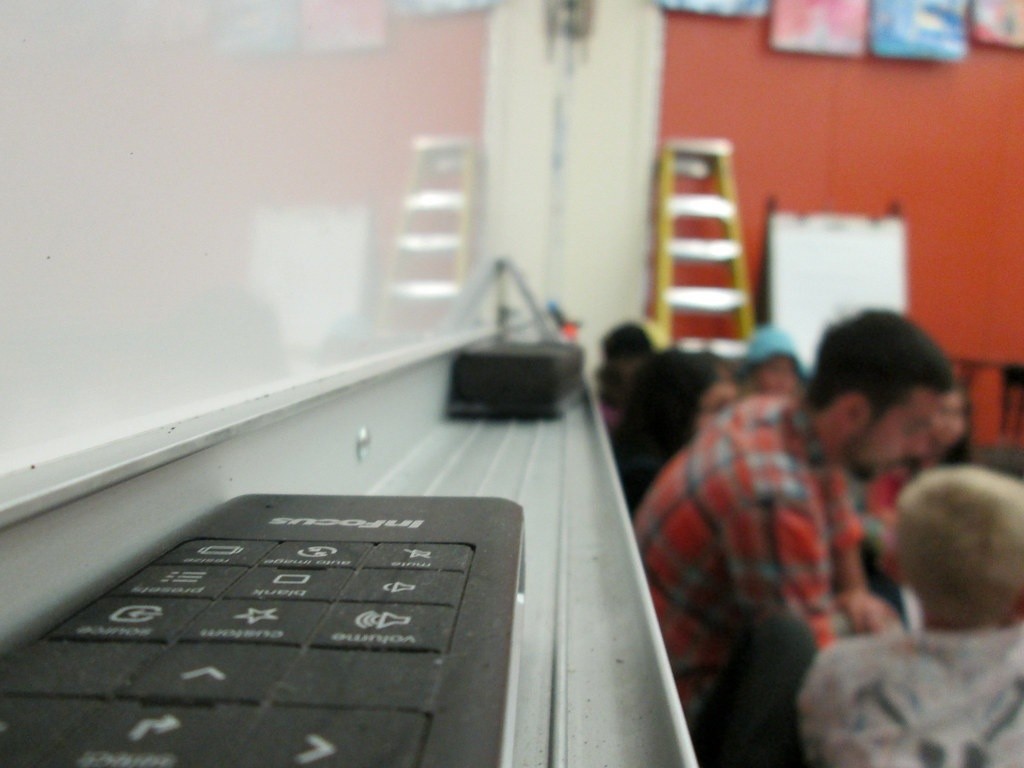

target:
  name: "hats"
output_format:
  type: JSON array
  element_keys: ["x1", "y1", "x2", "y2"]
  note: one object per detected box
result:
[{"x1": 745, "y1": 327, "x2": 803, "y2": 376}]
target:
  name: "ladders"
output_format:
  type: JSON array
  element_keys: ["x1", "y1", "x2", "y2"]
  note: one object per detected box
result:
[{"x1": 655, "y1": 137, "x2": 755, "y2": 339}]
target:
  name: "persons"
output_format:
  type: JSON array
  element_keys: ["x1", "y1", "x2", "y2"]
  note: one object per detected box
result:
[
  {"x1": 792, "y1": 466, "x2": 1024, "y2": 768},
  {"x1": 596, "y1": 307, "x2": 953, "y2": 730}
]
